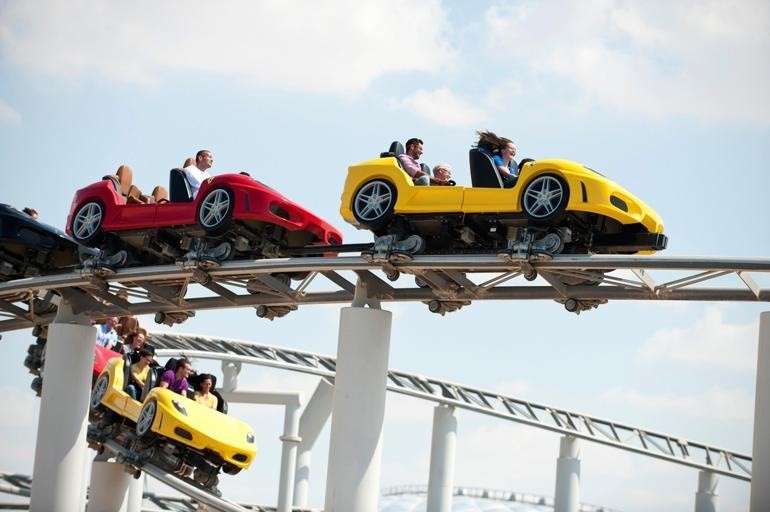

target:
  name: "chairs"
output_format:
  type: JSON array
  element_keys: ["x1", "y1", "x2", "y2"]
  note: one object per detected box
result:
[
  {"x1": 92, "y1": 316, "x2": 228, "y2": 413},
  {"x1": 147, "y1": 185, "x2": 168, "y2": 205},
  {"x1": 169, "y1": 157, "x2": 196, "y2": 203},
  {"x1": 381, "y1": 141, "x2": 404, "y2": 158},
  {"x1": 110, "y1": 164, "x2": 146, "y2": 205}
]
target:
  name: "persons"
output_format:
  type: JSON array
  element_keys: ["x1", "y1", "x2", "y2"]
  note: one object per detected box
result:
[
  {"x1": 23, "y1": 208, "x2": 38, "y2": 219},
  {"x1": 431, "y1": 164, "x2": 453, "y2": 184},
  {"x1": 398, "y1": 138, "x2": 449, "y2": 186},
  {"x1": 184, "y1": 149, "x2": 214, "y2": 201},
  {"x1": 90, "y1": 317, "x2": 218, "y2": 413}
]
